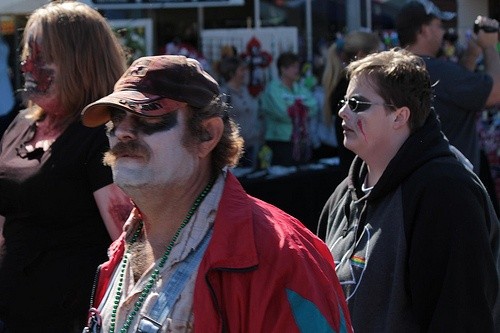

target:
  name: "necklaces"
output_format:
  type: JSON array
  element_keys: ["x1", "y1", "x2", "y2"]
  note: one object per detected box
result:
[{"x1": 108, "y1": 176, "x2": 219, "y2": 333}]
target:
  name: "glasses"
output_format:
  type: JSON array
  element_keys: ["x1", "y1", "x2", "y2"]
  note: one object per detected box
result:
[
  {"x1": 473, "y1": 22, "x2": 500, "y2": 35},
  {"x1": 338, "y1": 96, "x2": 399, "y2": 113},
  {"x1": 109, "y1": 106, "x2": 184, "y2": 134}
]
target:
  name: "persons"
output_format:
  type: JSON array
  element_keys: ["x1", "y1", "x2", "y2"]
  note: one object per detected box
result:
[
  {"x1": 80, "y1": 55, "x2": 355, "y2": 333},
  {"x1": 316, "y1": 46, "x2": 500, "y2": 333},
  {"x1": 321, "y1": 31, "x2": 381, "y2": 181},
  {"x1": 395, "y1": 0, "x2": 500, "y2": 145},
  {"x1": 165, "y1": 19, "x2": 338, "y2": 177},
  {"x1": 0, "y1": 0, "x2": 128, "y2": 333}
]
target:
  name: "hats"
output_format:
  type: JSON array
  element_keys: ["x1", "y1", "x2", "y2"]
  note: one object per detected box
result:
[
  {"x1": 81, "y1": 53, "x2": 222, "y2": 126},
  {"x1": 397, "y1": 0, "x2": 456, "y2": 27}
]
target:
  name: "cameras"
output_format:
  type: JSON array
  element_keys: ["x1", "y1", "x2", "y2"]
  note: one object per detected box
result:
[{"x1": 474, "y1": 19, "x2": 479, "y2": 33}]
